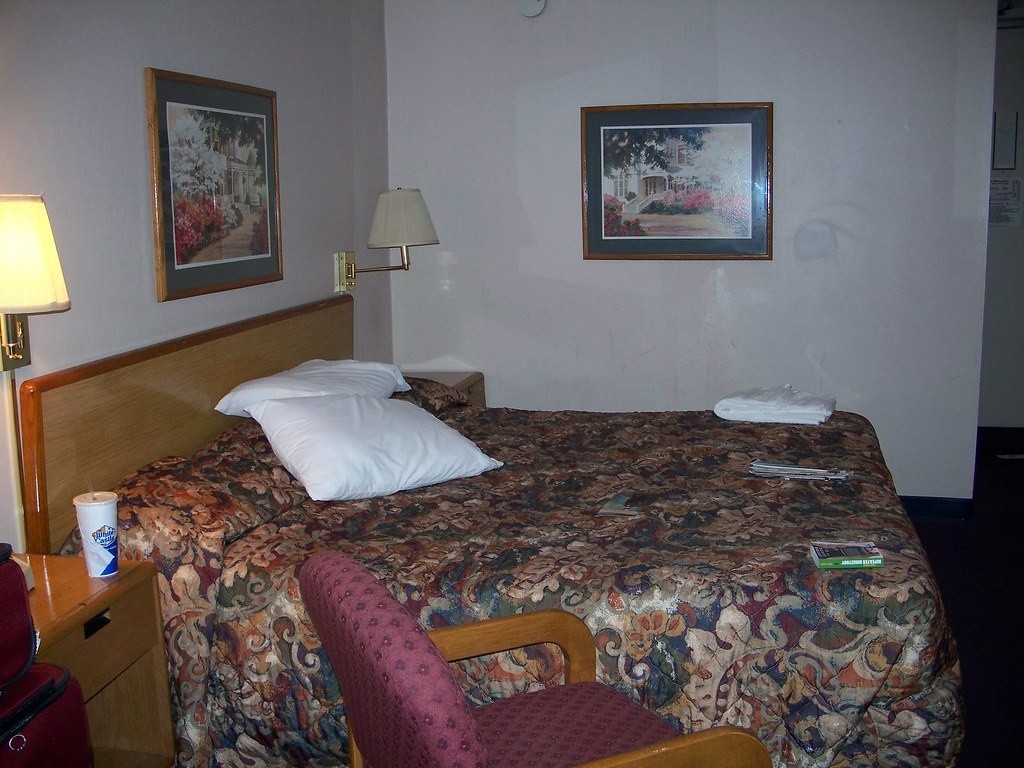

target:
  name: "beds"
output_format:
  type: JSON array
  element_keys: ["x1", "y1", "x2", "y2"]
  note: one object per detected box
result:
[{"x1": 20, "y1": 293, "x2": 967, "y2": 768}]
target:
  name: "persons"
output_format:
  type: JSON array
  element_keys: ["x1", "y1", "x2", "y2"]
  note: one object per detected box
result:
[{"x1": 813, "y1": 544, "x2": 847, "y2": 548}]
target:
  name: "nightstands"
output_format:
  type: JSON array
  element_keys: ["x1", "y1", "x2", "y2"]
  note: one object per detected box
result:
[
  {"x1": 10, "y1": 552, "x2": 176, "y2": 768},
  {"x1": 401, "y1": 371, "x2": 486, "y2": 407}
]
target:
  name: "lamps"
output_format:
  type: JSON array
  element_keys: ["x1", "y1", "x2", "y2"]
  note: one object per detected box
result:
[
  {"x1": 0, "y1": 194, "x2": 72, "y2": 373},
  {"x1": 338, "y1": 187, "x2": 440, "y2": 292}
]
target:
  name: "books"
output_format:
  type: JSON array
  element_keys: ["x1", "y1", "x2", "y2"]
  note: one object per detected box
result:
[{"x1": 810, "y1": 542, "x2": 884, "y2": 568}]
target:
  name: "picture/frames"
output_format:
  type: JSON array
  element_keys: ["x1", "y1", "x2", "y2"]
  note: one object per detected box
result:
[
  {"x1": 144, "y1": 67, "x2": 284, "y2": 303},
  {"x1": 581, "y1": 102, "x2": 773, "y2": 261}
]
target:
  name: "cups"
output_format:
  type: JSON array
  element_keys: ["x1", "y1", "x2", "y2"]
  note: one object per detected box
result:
[{"x1": 73, "y1": 491, "x2": 119, "y2": 578}]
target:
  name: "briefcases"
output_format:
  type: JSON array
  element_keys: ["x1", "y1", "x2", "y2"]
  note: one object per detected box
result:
[{"x1": 0, "y1": 542, "x2": 93, "y2": 768}]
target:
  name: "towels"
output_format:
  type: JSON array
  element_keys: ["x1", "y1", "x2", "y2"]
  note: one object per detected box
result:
[{"x1": 714, "y1": 384, "x2": 836, "y2": 425}]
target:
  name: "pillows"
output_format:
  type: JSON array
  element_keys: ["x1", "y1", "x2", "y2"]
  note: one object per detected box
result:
[
  {"x1": 243, "y1": 393, "x2": 504, "y2": 502},
  {"x1": 213, "y1": 358, "x2": 412, "y2": 418}
]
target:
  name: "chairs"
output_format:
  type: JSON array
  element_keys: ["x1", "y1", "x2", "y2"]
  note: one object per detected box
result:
[{"x1": 299, "y1": 550, "x2": 774, "y2": 768}]
